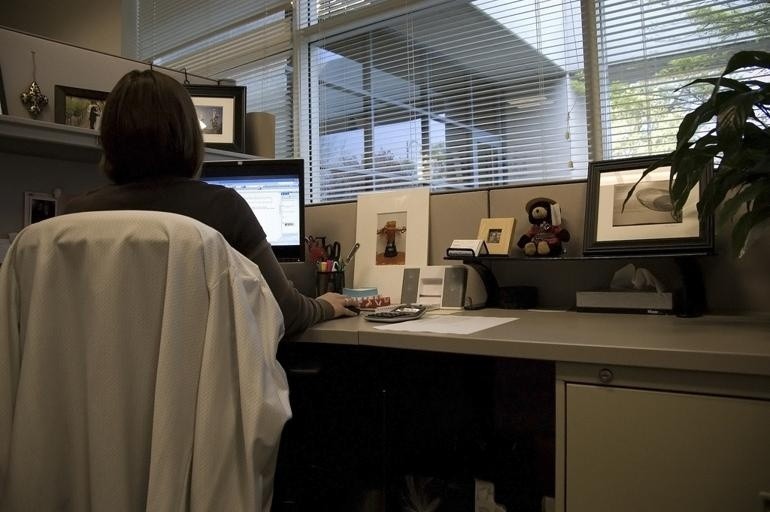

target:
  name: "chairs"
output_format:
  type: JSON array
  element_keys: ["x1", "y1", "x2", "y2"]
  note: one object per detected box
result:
[{"x1": 0, "y1": 209, "x2": 288, "y2": 511}]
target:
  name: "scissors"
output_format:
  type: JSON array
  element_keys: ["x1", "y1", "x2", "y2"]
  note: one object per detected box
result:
[{"x1": 324, "y1": 241, "x2": 340, "y2": 261}]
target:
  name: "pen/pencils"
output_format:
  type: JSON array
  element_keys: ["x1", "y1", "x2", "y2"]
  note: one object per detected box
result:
[{"x1": 316, "y1": 259, "x2": 346, "y2": 272}]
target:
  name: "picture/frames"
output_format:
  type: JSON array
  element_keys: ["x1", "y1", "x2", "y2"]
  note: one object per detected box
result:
[
  {"x1": 582, "y1": 153, "x2": 716, "y2": 256},
  {"x1": 53, "y1": 84, "x2": 112, "y2": 130},
  {"x1": 353, "y1": 187, "x2": 431, "y2": 304},
  {"x1": 181, "y1": 83, "x2": 247, "y2": 153},
  {"x1": 477, "y1": 217, "x2": 518, "y2": 255}
]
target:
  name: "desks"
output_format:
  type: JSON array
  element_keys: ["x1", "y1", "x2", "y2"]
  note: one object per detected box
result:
[{"x1": 295, "y1": 304, "x2": 770, "y2": 512}]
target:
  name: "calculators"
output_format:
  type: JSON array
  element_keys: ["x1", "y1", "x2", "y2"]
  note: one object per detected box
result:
[{"x1": 363, "y1": 305, "x2": 428, "y2": 323}]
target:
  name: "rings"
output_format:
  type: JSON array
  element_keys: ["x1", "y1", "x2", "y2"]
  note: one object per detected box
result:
[{"x1": 345, "y1": 298, "x2": 349, "y2": 307}]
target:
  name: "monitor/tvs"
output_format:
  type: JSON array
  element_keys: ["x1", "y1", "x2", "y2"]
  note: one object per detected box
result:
[{"x1": 200, "y1": 157, "x2": 305, "y2": 265}]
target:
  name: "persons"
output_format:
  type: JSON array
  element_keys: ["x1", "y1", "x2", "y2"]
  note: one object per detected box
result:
[
  {"x1": 63, "y1": 69, "x2": 361, "y2": 335},
  {"x1": 87, "y1": 100, "x2": 103, "y2": 130},
  {"x1": 88, "y1": 107, "x2": 99, "y2": 129}
]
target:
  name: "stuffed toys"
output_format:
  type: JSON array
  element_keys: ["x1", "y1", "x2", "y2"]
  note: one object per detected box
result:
[{"x1": 517, "y1": 197, "x2": 571, "y2": 257}]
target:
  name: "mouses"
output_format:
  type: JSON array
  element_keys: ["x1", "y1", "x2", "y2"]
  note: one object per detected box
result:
[{"x1": 341, "y1": 305, "x2": 360, "y2": 318}]
